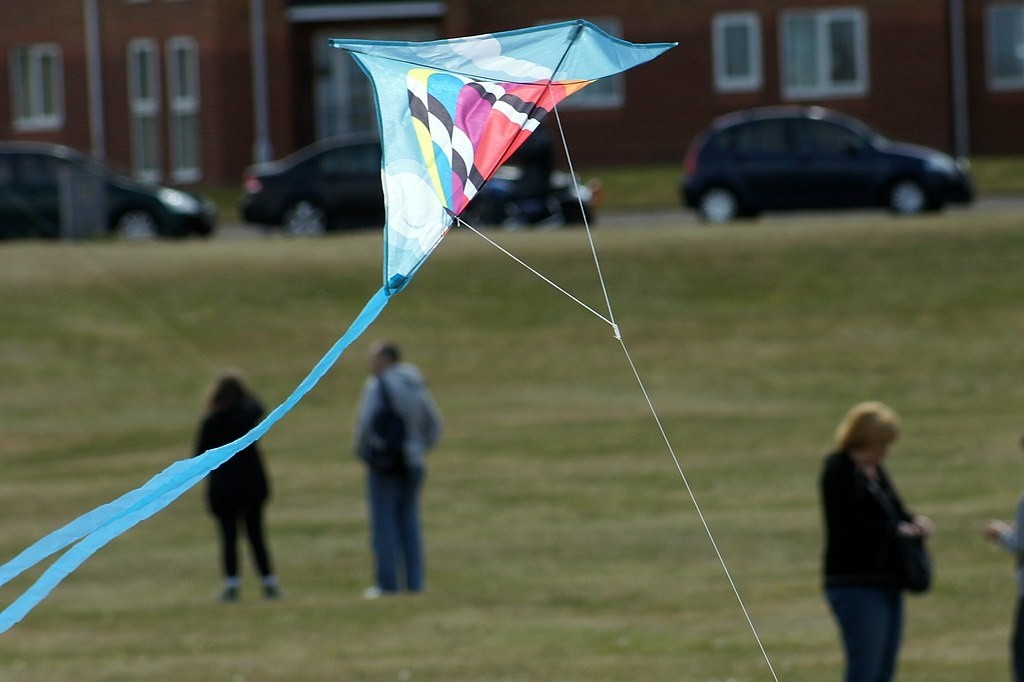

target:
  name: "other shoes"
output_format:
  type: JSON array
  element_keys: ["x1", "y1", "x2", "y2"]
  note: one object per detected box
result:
[
  {"x1": 216, "y1": 585, "x2": 241, "y2": 603},
  {"x1": 363, "y1": 585, "x2": 385, "y2": 601},
  {"x1": 260, "y1": 585, "x2": 282, "y2": 601}
]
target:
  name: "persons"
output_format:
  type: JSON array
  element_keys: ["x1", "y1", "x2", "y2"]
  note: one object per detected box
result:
[
  {"x1": 191, "y1": 375, "x2": 280, "y2": 600},
  {"x1": 985, "y1": 435, "x2": 1024, "y2": 681},
  {"x1": 354, "y1": 338, "x2": 444, "y2": 599},
  {"x1": 818, "y1": 402, "x2": 934, "y2": 682},
  {"x1": 502, "y1": 117, "x2": 565, "y2": 230}
]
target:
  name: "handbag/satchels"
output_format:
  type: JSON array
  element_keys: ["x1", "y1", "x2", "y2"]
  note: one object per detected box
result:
[
  {"x1": 361, "y1": 374, "x2": 411, "y2": 489},
  {"x1": 899, "y1": 535, "x2": 931, "y2": 593}
]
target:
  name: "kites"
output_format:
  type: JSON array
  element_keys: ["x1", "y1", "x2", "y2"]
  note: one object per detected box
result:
[{"x1": 0, "y1": 0, "x2": 677, "y2": 630}]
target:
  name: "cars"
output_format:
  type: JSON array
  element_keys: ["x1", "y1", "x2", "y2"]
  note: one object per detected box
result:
[
  {"x1": 678, "y1": 107, "x2": 976, "y2": 226},
  {"x1": 242, "y1": 134, "x2": 601, "y2": 234},
  {"x1": 1, "y1": 140, "x2": 215, "y2": 241}
]
